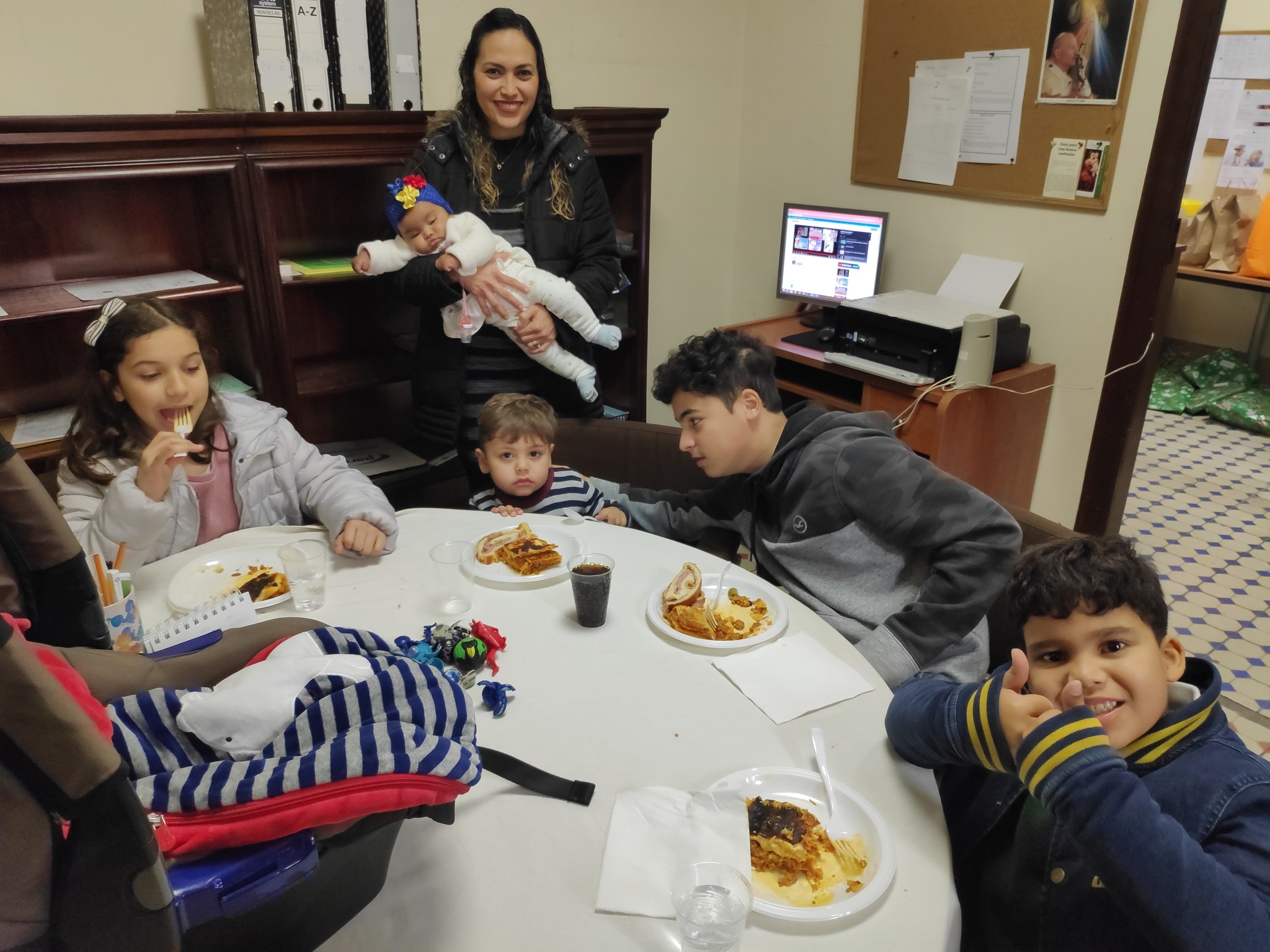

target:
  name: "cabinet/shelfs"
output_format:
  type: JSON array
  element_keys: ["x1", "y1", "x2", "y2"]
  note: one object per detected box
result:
[{"x1": 0, "y1": 107, "x2": 670, "y2": 468}]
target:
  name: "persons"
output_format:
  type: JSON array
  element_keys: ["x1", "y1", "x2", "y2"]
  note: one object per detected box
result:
[
  {"x1": 57, "y1": 295, "x2": 399, "y2": 559},
  {"x1": 594, "y1": 327, "x2": 1023, "y2": 692},
  {"x1": 352, "y1": 175, "x2": 623, "y2": 403},
  {"x1": 1040, "y1": 32, "x2": 1100, "y2": 100},
  {"x1": 466, "y1": 393, "x2": 634, "y2": 528},
  {"x1": 1076, "y1": 151, "x2": 1099, "y2": 192},
  {"x1": 387, "y1": 6, "x2": 623, "y2": 508},
  {"x1": 885, "y1": 531, "x2": 1270, "y2": 952}
]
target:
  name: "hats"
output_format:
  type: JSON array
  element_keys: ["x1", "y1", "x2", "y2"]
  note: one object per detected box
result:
[{"x1": 384, "y1": 173, "x2": 454, "y2": 239}]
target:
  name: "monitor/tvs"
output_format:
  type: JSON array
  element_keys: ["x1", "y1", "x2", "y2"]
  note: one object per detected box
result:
[{"x1": 776, "y1": 202, "x2": 889, "y2": 328}]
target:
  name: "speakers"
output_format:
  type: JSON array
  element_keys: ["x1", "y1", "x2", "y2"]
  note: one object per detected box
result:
[{"x1": 950, "y1": 314, "x2": 998, "y2": 390}]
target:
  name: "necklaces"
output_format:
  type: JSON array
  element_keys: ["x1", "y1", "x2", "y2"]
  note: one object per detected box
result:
[{"x1": 496, "y1": 148, "x2": 513, "y2": 170}]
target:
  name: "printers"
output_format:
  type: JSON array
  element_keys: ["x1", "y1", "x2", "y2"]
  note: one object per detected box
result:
[{"x1": 833, "y1": 253, "x2": 1031, "y2": 385}]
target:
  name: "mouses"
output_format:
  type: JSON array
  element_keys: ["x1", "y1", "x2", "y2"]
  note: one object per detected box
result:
[{"x1": 817, "y1": 326, "x2": 836, "y2": 343}]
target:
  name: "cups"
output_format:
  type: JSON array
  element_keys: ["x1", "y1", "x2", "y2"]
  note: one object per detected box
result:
[
  {"x1": 277, "y1": 539, "x2": 327, "y2": 611},
  {"x1": 102, "y1": 585, "x2": 145, "y2": 653},
  {"x1": 670, "y1": 861, "x2": 754, "y2": 952},
  {"x1": 429, "y1": 541, "x2": 479, "y2": 615},
  {"x1": 566, "y1": 553, "x2": 615, "y2": 628}
]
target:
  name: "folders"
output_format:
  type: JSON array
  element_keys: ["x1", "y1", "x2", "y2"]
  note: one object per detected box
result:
[
  {"x1": 247, "y1": 0, "x2": 298, "y2": 113},
  {"x1": 372, "y1": 1, "x2": 423, "y2": 109},
  {"x1": 324, "y1": 0, "x2": 373, "y2": 110},
  {"x1": 288, "y1": 0, "x2": 340, "y2": 112}
]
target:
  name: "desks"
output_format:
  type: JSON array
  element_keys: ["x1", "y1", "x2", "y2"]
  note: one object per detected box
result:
[
  {"x1": 719, "y1": 311, "x2": 1056, "y2": 510},
  {"x1": 1177, "y1": 265, "x2": 1270, "y2": 366},
  {"x1": 133, "y1": 508, "x2": 963, "y2": 952}
]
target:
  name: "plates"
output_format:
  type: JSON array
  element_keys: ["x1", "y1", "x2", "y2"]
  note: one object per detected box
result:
[
  {"x1": 168, "y1": 544, "x2": 305, "y2": 614},
  {"x1": 705, "y1": 767, "x2": 897, "y2": 921},
  {"x1": 647, "y1": 573, "x2": 789, "y2": 649},
  {"x1": 461, "y1": 525, "x2": 587, "y2": 583}
]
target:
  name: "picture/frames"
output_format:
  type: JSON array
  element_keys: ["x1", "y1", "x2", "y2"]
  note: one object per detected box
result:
[{"x1": 1037, "y1": 0, "x2": 1136, "y2": 105}]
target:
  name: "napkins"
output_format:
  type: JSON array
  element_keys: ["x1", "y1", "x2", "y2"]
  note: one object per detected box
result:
[
  {"x1": 594, "y1": 787, "x2": 693, "y2": 918},
  {"x1": 711, "y1": 633, "x2": 874, "y2": 725}
]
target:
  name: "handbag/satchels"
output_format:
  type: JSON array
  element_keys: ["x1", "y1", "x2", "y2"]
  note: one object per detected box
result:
[
  {"x1": 1180, "y1": 179, "x2": 1260, "y2": 272},
  {"x1": 1238, "y1": 192, "x2": 1270, "y2": 279}
]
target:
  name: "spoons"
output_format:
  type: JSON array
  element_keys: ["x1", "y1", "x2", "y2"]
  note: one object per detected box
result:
[{"x1": 563, "y1": 507, "x2": 607, "y2": 523}]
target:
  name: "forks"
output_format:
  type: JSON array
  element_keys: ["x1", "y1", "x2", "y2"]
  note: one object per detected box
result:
[
  {"x1": 812, "y1": 727, "x2": 865, "y2": 862},
  {"x1": 174, "y1": 406, "x2": 193, "y2": 457},
  {"x1": 703, "y1": 561, "x2": 733, "y2": 635}
]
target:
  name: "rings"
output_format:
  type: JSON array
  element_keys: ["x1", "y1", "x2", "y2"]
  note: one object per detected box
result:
[{"x1": 532, "y1": 339, "x2": 538, "y2": 347}]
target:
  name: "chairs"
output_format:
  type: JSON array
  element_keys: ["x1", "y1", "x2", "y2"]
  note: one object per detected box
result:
[{"x1": 551, "y1": 418, "x2": 727, "y2": 494}]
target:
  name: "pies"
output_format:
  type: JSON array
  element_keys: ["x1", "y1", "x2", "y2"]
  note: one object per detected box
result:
[
  {"x1": 661, "y1": 561, "x2": 716, "y2": 639},
  {"x1": 476, "y1": 523, "x2": 562, "y2": 577},
  {"x1": 743, "y1": 796, "x2": 821, "y2": 872},
  {"x1": 230, "y1": 568, "x2": 288, "y2": 603}
]
